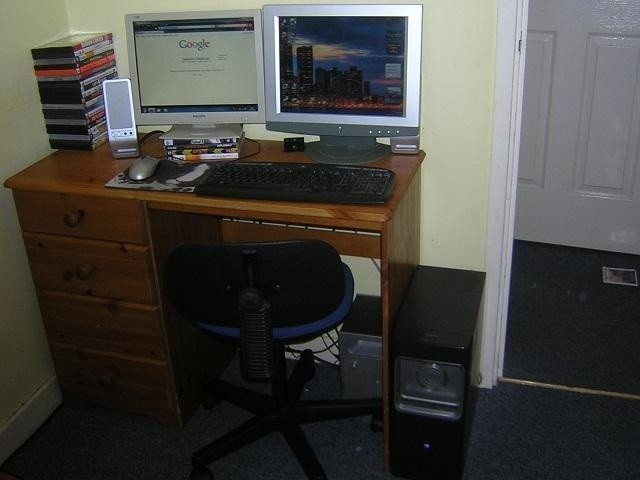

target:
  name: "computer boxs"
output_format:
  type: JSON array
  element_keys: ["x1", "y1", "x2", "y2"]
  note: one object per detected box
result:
[{"x1": 391, "y1": 264, "x2": 486, "y2": 480}]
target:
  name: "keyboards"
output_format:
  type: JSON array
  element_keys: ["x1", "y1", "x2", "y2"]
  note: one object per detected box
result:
[{"x1": 195, "y1": 161, "x2": 395, "y2": 204}]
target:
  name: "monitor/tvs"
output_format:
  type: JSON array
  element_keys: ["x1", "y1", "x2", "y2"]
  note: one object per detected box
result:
[
  {"x1": 262, "y1": 4, "x2": 424, "y2": 166},
  {"x1": 124, "y1": 8, "x2": 266, "y2": 140}
]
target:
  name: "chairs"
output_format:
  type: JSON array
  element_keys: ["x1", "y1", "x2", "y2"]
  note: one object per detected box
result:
[{"x1": 163, "y1": 239, "x2": 386, "y2": 480}]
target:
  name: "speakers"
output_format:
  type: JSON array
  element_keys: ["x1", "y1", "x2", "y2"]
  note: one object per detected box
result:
[
  {"x1": 101, "y1": 77, "x2": 143, "y2": 159},
  {"x1": 390, "y1": 134, "x2": 420, "y2": 156}
]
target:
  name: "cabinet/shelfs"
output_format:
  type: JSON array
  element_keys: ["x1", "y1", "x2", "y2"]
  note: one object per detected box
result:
[{"x1": 4, "y1": 129, "x2": 426, "y2": 479}]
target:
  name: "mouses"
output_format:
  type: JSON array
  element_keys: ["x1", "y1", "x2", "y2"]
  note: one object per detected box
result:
[{"x1": 130, "y1": 156, "x2": 160, "y2": 180}]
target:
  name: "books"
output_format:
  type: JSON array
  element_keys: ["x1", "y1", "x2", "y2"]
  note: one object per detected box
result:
[
  {"x1": 31, "y1": 30, "x2": 118, "y2": 153},
  {"x1": 162, "y1": 123, "x2": 246, "y2": 163}
]
